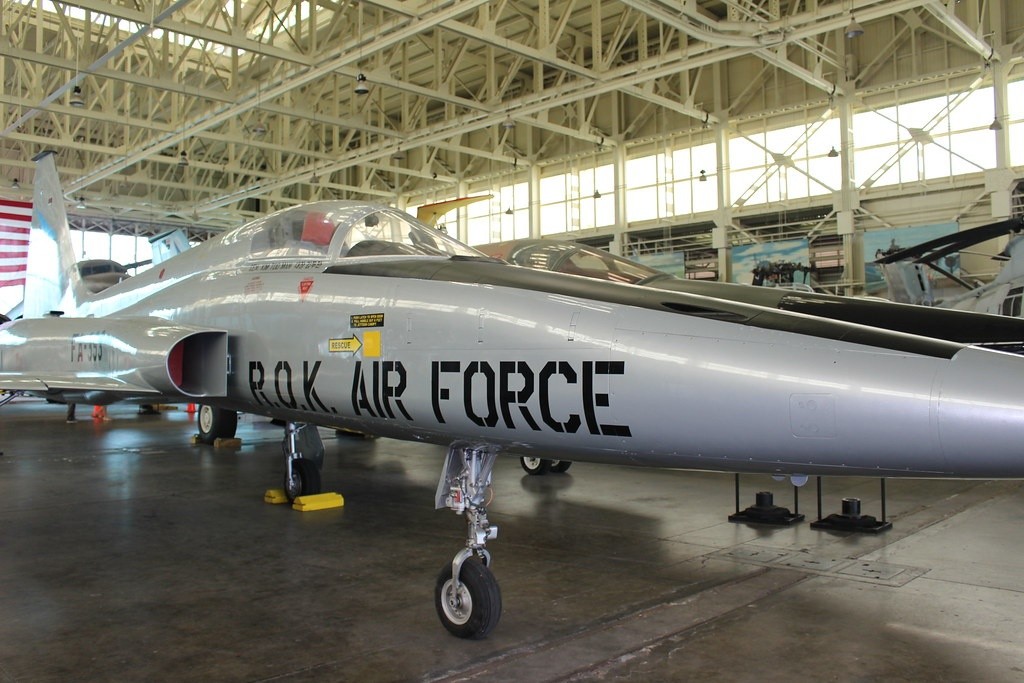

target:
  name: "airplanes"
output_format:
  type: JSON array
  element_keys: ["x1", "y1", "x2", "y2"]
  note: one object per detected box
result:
[
  {"x1": 0, "y1": 155, "x2": 1024, "y2": 642},
  {"x1": 2, "y1": 257, "x2": 157, "y2": 416}
]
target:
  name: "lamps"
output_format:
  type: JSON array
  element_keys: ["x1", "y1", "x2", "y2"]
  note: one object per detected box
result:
[
  {"x1": 502, "y1": 63, "x2": 1002, "y2": 215},
  {"x1": 8, "y1": 178, "x2": 21, "y2": 191},
  {"x1": 75, "y1": 197, "x2": 86, "y2": 209},
  {"x1": 68, "y1": 1, "x2": 85, "y2": 107},
  {"x1": 844, "y1": 1, "x2": 864, "y2": 39},
  {"x1": 352, "y1": 0, "x2": 370, "y2": 94}
]
